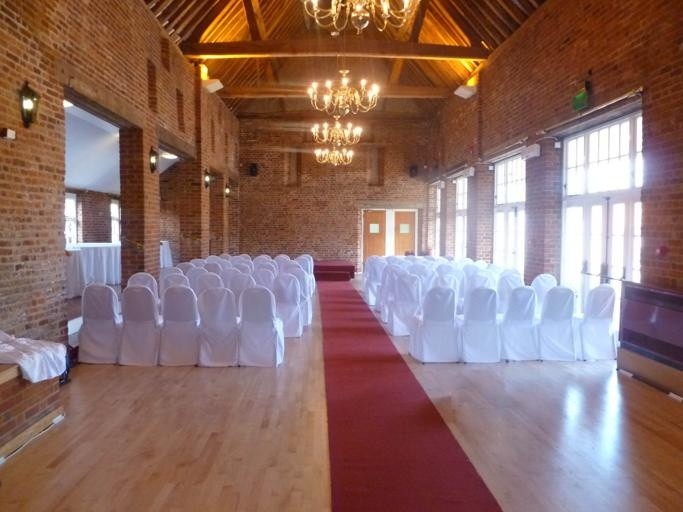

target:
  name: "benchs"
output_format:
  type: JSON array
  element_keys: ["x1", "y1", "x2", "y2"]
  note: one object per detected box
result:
[{"x1": 0, "y1": 347, "x2": 66, "y2": 466}]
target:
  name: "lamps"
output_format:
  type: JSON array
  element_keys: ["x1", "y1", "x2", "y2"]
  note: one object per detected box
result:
[
  {"x1": 314, "y1": 57, "x2": 355, "y2": 167},
  {"x1": 202, "y1": 77, "x2": 223, "y2": 94},
  {"x1": 310, "y1": 54, "x2": 363, "y2": 146},
  {"x1": 226, "y1": 184, "x2": 230, "y2": 198},
  {"x1": 306, "y1": 28, "x2": 382, "y2": 115},
  {"x1": 454, "y1": 84, "x2": 476, "y2": 100},
  {"x1": 19, "y1": 80, "x2": 41, "y2": 129},
  {"x1": 205, "y1": 168, "x2": 211, "y2": 187},
  {"x1": 303, "y1": 0, "x2": 423, "y2": 39},
  {"x1": 149, "y1": 148, "x2": 159, "y2": 173}
]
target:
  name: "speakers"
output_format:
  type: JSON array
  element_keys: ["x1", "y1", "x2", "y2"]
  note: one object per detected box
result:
[
  {"x1": 249, "y1": 163, "x2": 258, "y2": 176},
  {"x1": 410, "y1": 165, "x2": 418, "y2": 177}
]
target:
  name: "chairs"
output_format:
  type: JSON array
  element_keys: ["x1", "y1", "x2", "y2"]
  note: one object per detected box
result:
[
  {"x1": 361, "y1": 253, "x2": 619, "y2": 365},
  {"x1": 75, "y1": 251, "x2": 317, "y2": 369}
]
